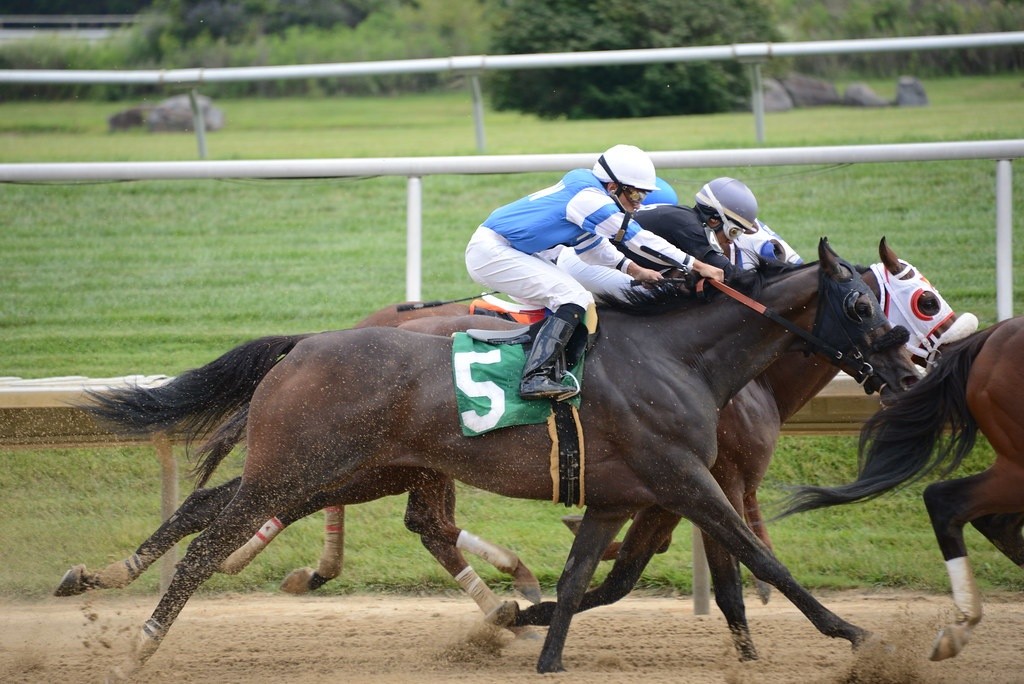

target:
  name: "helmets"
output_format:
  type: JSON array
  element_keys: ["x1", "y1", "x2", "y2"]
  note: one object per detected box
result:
[
  {"x1": 591, "y1": 143, "x2": 662, "y2": 192},
  {"x1": 695, "y1": 176, "x2": 760, "y2": 230}
]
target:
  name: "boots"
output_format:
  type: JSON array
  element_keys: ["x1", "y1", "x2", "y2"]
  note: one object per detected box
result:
[{"x1": 516, "y1": 314, "x2": 581, "y2": 400}]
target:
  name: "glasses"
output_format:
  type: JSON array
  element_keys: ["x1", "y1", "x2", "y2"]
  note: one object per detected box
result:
[
  {"x1": 701, "y1": 183, "x2": 744, "y2": 239},
  {"x1": 596, "y1": 154, "x2": 647, "y2": 206}
]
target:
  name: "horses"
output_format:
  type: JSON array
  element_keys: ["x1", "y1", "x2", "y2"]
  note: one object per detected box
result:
[
  {"x1": 54, "y1": 237, "x2": 924, "y2": 684},
  {"x1": 217, "y1": 234, "x2": 807, "y2": 605},
  {"x1": 182, "y1": 234, "x2": 979, "y2": 638},
  {"x1": 740, "y1": 316, "x2": 1024, "y2": 662}
]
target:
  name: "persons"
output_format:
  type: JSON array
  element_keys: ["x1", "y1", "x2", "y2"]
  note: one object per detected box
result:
[
  {"x1": 511, "y1": 177, "x2": 760, "y2": 305},
  {"x1": 463, "y1": 145, "x2": 721, "y2": 398}
]
